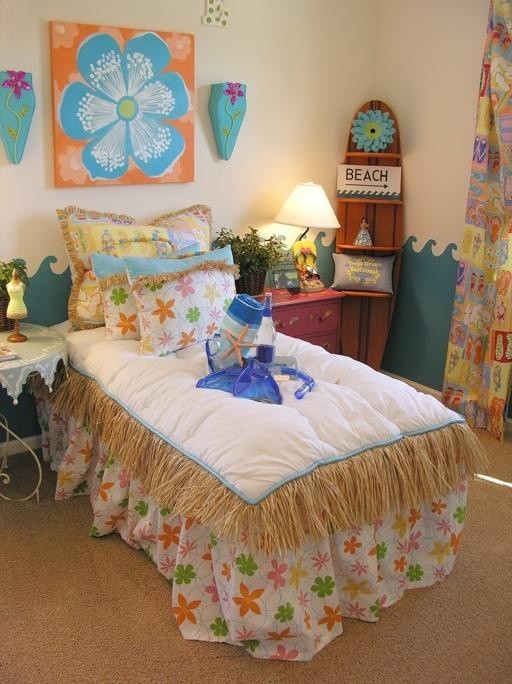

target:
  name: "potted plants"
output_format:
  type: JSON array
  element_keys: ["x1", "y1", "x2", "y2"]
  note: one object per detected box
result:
[
  {"x1": 212, "y1": 226, "x2": 290, "y2": 295},
  {"x1": 0, "y1": 258, "x2": 31, "y2": 332}
]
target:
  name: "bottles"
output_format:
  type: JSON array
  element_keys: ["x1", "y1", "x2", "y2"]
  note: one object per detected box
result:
[{"x1": 256, "y1": 292, "x2": 276, "y2": 364}]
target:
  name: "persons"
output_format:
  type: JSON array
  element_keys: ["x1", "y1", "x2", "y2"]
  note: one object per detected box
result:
[{"x1": 352, "y1": 216, "x2": 374, "y2": 245}]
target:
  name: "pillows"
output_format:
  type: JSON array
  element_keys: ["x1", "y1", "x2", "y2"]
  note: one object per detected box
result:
[
  {"x1": 89, "y1": 242, "x2": 200, "y2": 341},
  {"x1": 56, "y1": 204, "x2": 212, "y2": 334},
  {"x1": 330, "y1": 252, "x2": 396, "y2": 294},
  {"x1": 123, "y1": 245, "x2": 237, "y2": 357}
]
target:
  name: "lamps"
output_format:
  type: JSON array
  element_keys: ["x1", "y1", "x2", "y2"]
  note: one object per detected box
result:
[
  {"x1": 6, "y1": 268, "x2": 28, "y2": 343},
  {"x1": 274, "y1": 181, "x2": 341, "y2": 261}
]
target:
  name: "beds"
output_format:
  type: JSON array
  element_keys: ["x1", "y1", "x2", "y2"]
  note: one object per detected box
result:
[{"x1": 36, "y1": 318, "x2": 491, "y2": 661}]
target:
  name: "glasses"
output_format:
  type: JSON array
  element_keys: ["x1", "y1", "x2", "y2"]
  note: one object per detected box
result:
[{"x1": 206, "y1": 339, "x2": 221, "y2": 377}]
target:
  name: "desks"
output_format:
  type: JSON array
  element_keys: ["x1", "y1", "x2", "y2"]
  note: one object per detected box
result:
[{"x1": 0, "y1": 323, "x2": 70, "y2": 502}]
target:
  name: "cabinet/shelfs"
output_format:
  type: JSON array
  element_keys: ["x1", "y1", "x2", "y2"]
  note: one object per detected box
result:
[
  {"x1": 249, "y1": 287, "x2": 342, "y2": 354},
  {"x1": 336, "y1": 100, "x2": 404, "y2": 372}
]
